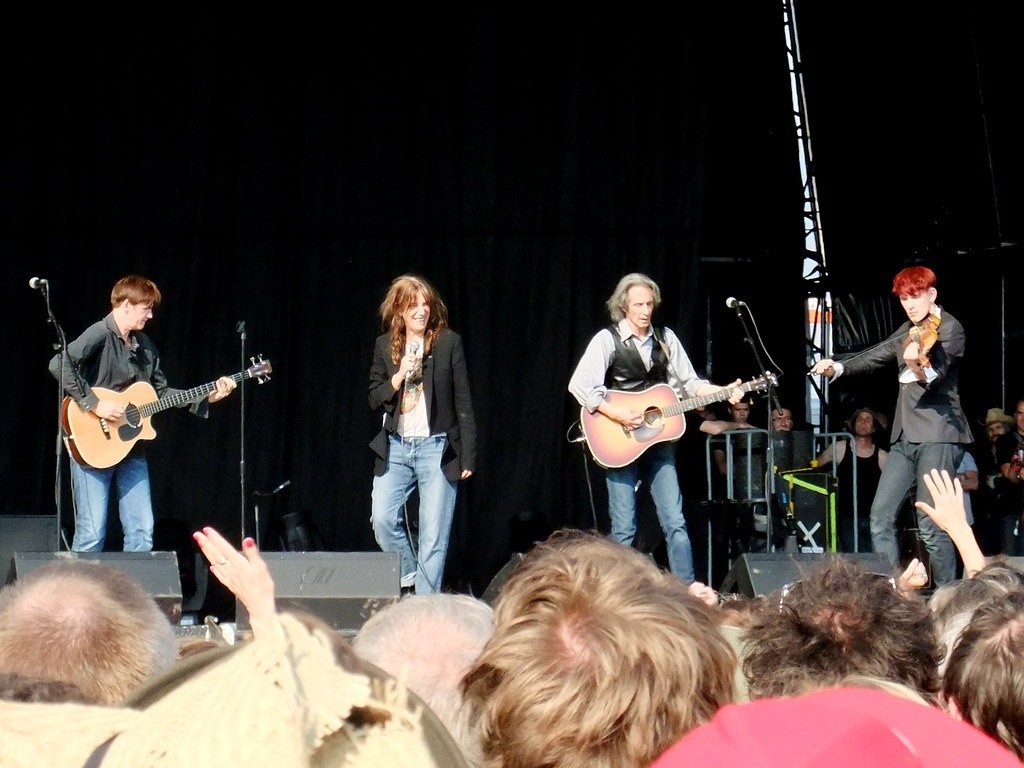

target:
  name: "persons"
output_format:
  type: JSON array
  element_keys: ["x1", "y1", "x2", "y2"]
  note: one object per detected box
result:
[
  {"x1": 1, "y1": 466, "x2": 1024, "y2": 767},
  {"x1": 1008, "y1": 437, "x2": 1024, "y2": 557},
  {"x1": 812, "y1": 265, "x2": 977, "y2": 587},
  {"x1": 713, "y1": 396, "x2": 752, "y2": 476},
  {"x1": 973, "y1": 408, "x2": 1016, "y2": 557},
  {"x1": 370, "y1": 277, "x2": 479, "y2": 595},
  {"x1": 567, "y1": 274, "x2": 746, "y2": 590},
  {"x1": 815, "y1": 406, "x2": 889, "y2": 553},
  {"x1": 997, "y1": 400, "x2": 1024, "y2": 557},
  {"x1": 655, "y1": 405, "x2": 759, "y2": 569},
  {"x1": 769, "y1": 408, "x2": 793, "y2": 432},
  {"x1": 49, "y1": 277, "x2": 238, "y2": 551},
  {"x1": 953, "y1": 452, "x2": 980, "y2": 580}
]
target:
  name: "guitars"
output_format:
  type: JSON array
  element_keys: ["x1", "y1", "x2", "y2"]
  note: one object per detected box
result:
[
  {"x1": 63, "y1": 353, "x2": 274, "y2": 469},
  {"x1": 581, "y1": 370, "x2": 779, "y2": 469}
]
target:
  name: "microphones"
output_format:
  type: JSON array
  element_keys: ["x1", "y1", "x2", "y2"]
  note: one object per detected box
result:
[
  {"x1": 726, "y1": 297, "x2": 742, "y2": 308},
  {"x1": 406, "y1": 341, "x2": 420, "y2": 378},
  {"x1": 29, "y1": 277, "x2": 47, "y2": 289}
]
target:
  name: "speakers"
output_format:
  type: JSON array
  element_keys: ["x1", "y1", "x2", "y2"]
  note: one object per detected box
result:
[
  {"x1": 719, "y1": 552, "x2": 894, "y2": 599},
  {"x1": 0, "y1": 513, "x2": 183, "y2": 628},
  {"x1": 236, "y1": 551, "x2": 400, "y2": 630}
]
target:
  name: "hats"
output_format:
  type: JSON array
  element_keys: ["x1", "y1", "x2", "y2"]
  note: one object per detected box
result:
[{"x1": 978, "y1": 408, "x2": 1015, "y2": 425}]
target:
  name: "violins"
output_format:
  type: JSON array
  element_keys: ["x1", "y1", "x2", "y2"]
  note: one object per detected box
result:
[{"x1": 902, "y1": 305, "x2": 943, "y2": 384}]
